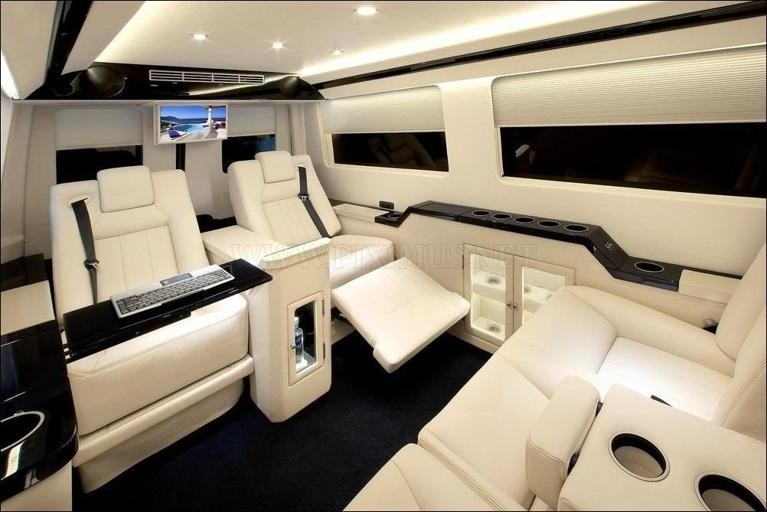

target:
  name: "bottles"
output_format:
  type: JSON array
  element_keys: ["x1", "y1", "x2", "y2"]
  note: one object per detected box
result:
[{"x1": 294, "y1": 316, "x2": 304, "y2": 364}]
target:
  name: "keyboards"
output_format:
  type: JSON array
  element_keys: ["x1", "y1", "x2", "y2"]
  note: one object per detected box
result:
[{"x1": 110, "y1": 263, "x2": 235, "y2": 319}]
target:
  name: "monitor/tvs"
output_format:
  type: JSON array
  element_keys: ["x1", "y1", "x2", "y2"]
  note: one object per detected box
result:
[{"x1": 152, "y1": 104, "x2": 228, "y2": 145}]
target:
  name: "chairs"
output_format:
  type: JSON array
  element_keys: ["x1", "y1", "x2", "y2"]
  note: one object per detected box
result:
[
  {"x1": 50, "y1": 166, "x2": 255, "y2": 493},
  {"x1": 227, "y1": 150, "x2": 395, "y2": 345}
]
